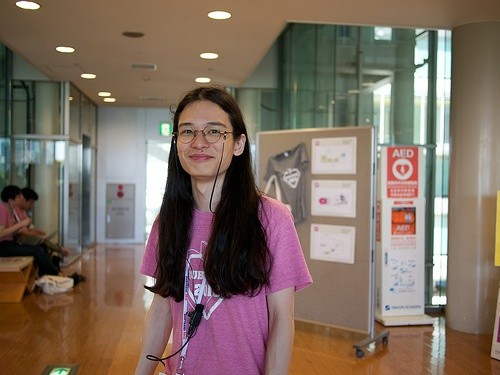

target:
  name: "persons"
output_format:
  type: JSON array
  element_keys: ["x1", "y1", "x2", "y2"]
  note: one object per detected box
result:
[
  {"x1": 14, "y1": 187, "x2": 69, "y2": 256},
  {"x1": 133, "y1": 84, "x2": 312, "y2": 375},
  {"x1": 0, "y1": 184, "x2": 81, "y2": 289}
]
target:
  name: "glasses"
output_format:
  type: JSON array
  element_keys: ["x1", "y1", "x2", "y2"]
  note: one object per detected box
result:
[{"x1": 171, "y1": 126, "x2": 229, "y2": 144}]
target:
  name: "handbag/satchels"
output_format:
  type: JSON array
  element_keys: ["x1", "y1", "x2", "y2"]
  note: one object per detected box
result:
[
  {"x1": 13, "y1": 230, "x2": 44, "y2": 246},
  {"x1": 34, "y1": 274, "x2": 74, "y2": 294}
]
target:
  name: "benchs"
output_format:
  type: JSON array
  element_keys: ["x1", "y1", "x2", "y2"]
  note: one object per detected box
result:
[{"x1": 0, "y1": 256, "x2": 42, "y2": 301}]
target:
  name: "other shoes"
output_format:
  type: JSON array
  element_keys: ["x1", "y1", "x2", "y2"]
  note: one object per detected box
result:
[{"x1": 67, "y1": 271, "x2": 79, "y2": 287}]
